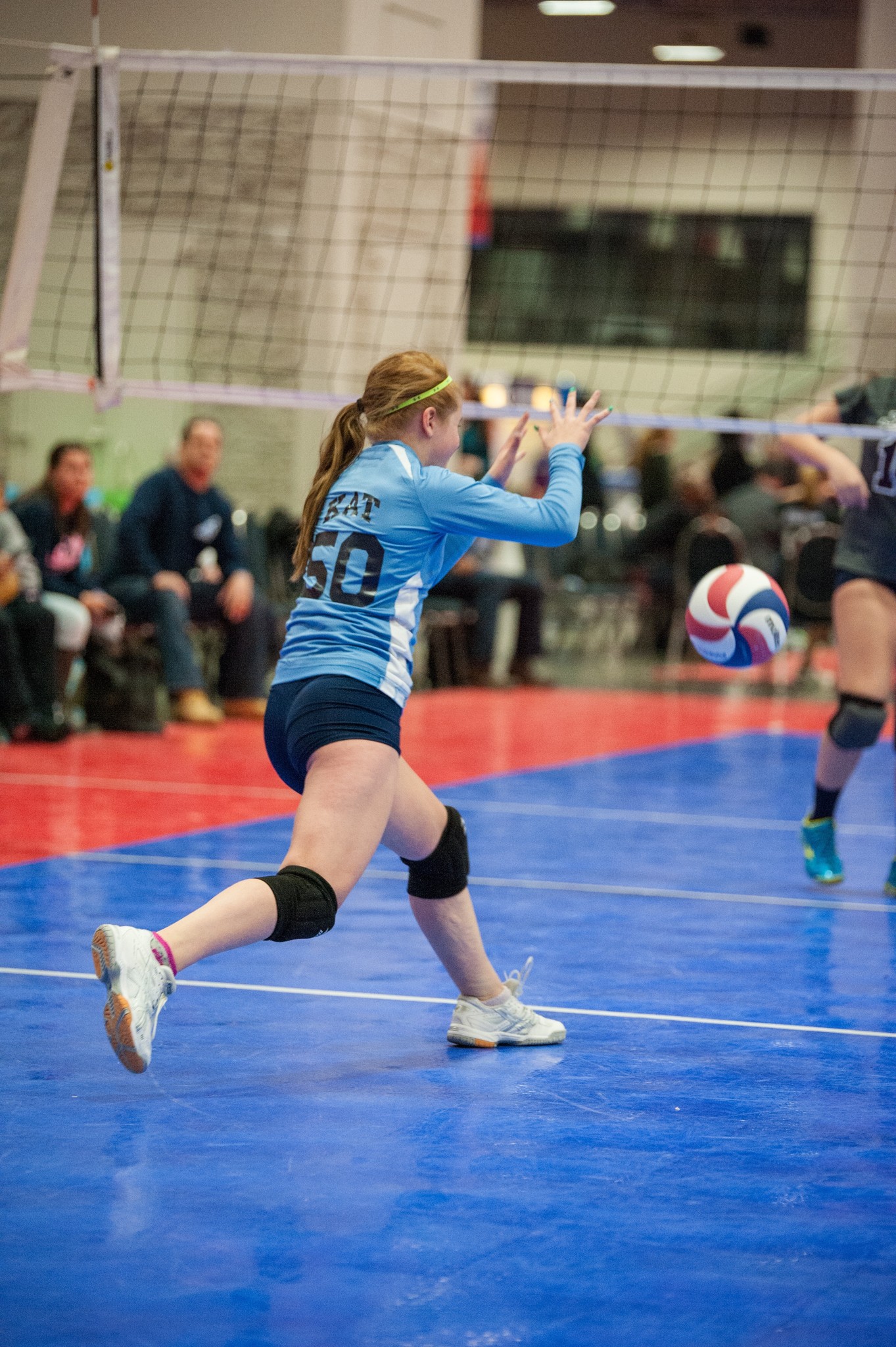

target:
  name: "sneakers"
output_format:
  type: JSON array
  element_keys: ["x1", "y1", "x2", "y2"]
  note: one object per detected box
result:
[
  {"x1": 800, "y1": 808, "x2": 844, "y2": 883},
  {"x1": 92, "y1": 924, "x2": 176, "y2": 1073},
  {"x1": 447, "y1": 978, "x2": 566, "y2": 1047},
  {"x1": 883, "y1": 855, "x2": 896, "y2": 898}
]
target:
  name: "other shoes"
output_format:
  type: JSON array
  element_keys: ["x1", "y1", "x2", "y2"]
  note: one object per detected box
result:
[
  {"x1": 510, "y1": 660, "x2": 552, "y2": 685},
  {"x1": 219, "y1": 697, "x2": 269, "y2": 718},
  {"x1": 175, "y1": 698, "x2": 222, "y2": 722},
  {"x1": 456, "y1": 658, "x2": 488, "y2": 687}
]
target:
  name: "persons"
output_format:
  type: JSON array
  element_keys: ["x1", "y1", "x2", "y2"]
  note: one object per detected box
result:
[
  {"x1": 93, "y1": 352, "x2": 611, "y2": 1072},
  {"x1": 1, "y1": 342, "x2": 895, "y2": 896}
]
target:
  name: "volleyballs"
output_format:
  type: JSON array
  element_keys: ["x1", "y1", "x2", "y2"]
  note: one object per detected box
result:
[{"x1": 684, "y1": 563, "x2": 790, "y2": 669}]
